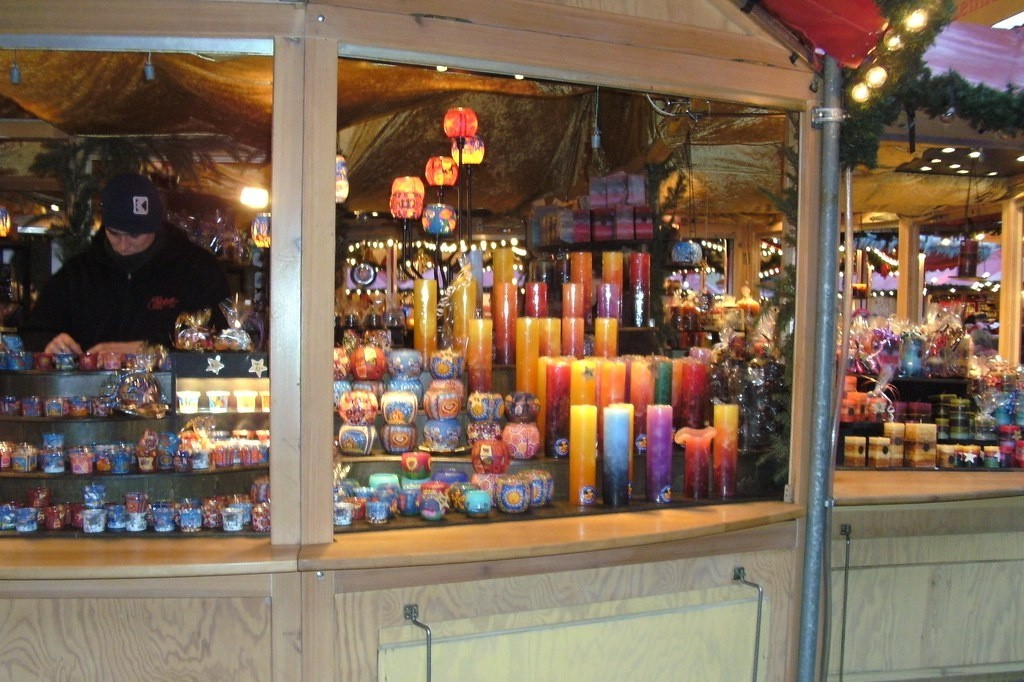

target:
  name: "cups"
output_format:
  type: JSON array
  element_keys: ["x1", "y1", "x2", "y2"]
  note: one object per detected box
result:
[
  {"x1": 841, "y1": 376, "x2": 1024, "y2": 469},
  {"x1": 90, "y1": 397, "x2": 112, "y2": 416},
  {"x1": 177, "y1": 391, "x2": 201, "y2": 413},
  {"x1": 0, "y1": 350, "x2": 33, "y2": 370},
  {"x1": 45, "y1": 397, "x2": 64, "y2": 417},
  {"x1": 21, "y1": 397, "x2": 42, "y2": 416},
  {"x1": 333, "y1": 452, "x2": 448, "y2": 525},
  {"x1": 68, "y1": 397, "x2": 89, "y2": 416},
  {"x1": 260, "y1": 391, "x2": 270, "y2": 412},
  {"x1": 55, "y1": 353, "x2": 75, "y2": 372},
  {"x1": 125, "y1": 354, "x2": 138, "y2": 368},
  {"x1": 37, "y1": 353, "x2": 52, "y2": 370},
  {"x1": 0, "y1": 479, "x2": 271, "y2": 533},
  {"x1": 102, "y1": 353, "x2": 121, "y2": 370},
  {"x1": 206, "y1": 391, "x2": 230, "y2": 413},
  {"x1": 78, "y1": 353, "x2": 98, "y2": 370},
  {"x1": 234, "y1": 390, "x2": 258, "y2": 413},
  {"x1": 0, "y1": 429, "x2": 269, "y2": 475},
  {"x1": 0, "y1": 396, "x2": 21, "y2": 416}
]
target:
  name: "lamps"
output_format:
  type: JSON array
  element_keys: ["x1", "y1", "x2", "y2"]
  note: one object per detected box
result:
[
  {"x1": 335, "y1": 153, "x2": 349, "y2": 178},
  {"x1": 444, "y1": 106, "x2": 477, "y2": 139},
  {"x1": 424, "y1": 154, "x2": 459, "y2": 188},
  {"x1": 449, "y1": 135, "x2": 486, "y2": 167},
  {"x1": 390, "y1": 175, "x2": 425, "y2": 200},
  {"x1": 863, "y1": 64, "x2": 888, "y2": 89},
  {"x1": 904, "y1": 8, "x2": 932, "y2": 33},
  {"x1": 421, "y1": 202, "x2": 457, "y2": 236},
  {"x1": 0, "y1": 205, "x2": 11, "y2": 239},
  {"x1": 250, "y1": 212, "x2": 271, "y2": 249},
  {"x1": 334, "y1": 170, "x2": 349, "y2": 202},
  {"x1": 882, "y1": 25, "x2": 906, "y2": 52},
  {"x1": 389, "y1": 188, "x2": 422, "y2": 218},
  {"x1": 850, "y1": 79, "x2": 872, "y2": 103}
]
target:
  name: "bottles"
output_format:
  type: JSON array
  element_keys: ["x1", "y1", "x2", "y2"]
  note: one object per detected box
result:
[
  {"x1": 384, "y1": 313, "x2": 404, "y2": 351},
  {"x1": 342, "y1": 315, "x2": 360, "y2": 353},
  {"x1": 364, "y1": 314, "x2": 387, "y2": 352}
]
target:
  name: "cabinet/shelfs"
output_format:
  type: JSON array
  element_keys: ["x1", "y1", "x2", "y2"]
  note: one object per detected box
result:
[
  {"x1": 833, "y1": 357, "x2": 1024, "y2": 472},
  {"x1": 1, "y1": 328, "x2": 730, "y2": 533},
  {"x1": 925, "y1": 287, "x2": 1000, "y2": 336}
]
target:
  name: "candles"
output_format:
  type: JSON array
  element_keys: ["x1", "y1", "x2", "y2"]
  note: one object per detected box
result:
[{"x1": 412, "y1": 246, "x2": 742, "y2": 509}]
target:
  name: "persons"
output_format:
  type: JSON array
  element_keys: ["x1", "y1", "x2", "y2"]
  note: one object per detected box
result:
[{"x1": 16, "y1": 172, "x2": 231, "y2": 366}]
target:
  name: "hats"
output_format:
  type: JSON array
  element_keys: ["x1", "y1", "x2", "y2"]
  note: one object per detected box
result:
[{"x1": 101, "y1": 173, "x2": 163, "y2": 233}]
